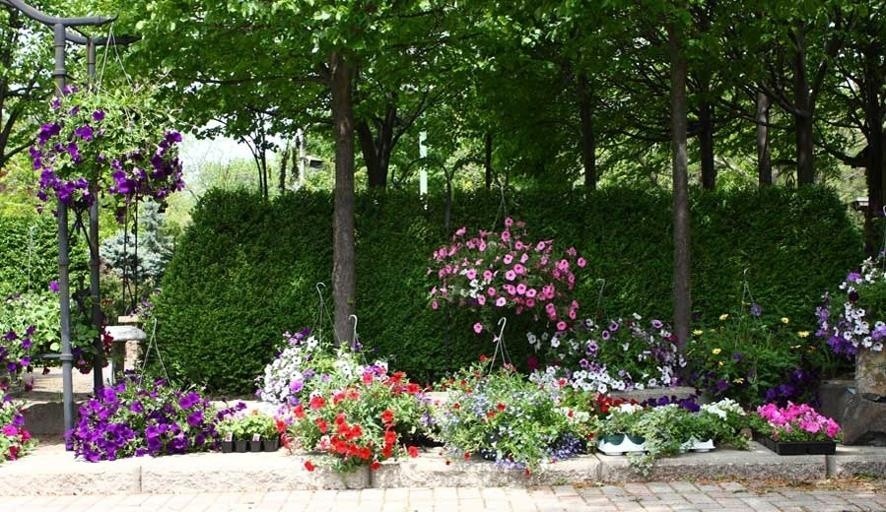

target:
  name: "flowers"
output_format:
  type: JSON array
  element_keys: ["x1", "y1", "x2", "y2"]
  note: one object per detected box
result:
[
  {"x1": 256, "y1": 327, "x2": 432, "y2": 474},
  {"x1": 34, "y1": 20, "x2": 185, "y2": 214},
  {"x1": 1, "y1": 298, "x2": 213, "y2": 463}
]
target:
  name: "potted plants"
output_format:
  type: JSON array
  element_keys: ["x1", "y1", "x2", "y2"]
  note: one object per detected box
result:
[{"x1": 215, "y1": 412, "x2": 285, "y2": 453}]
